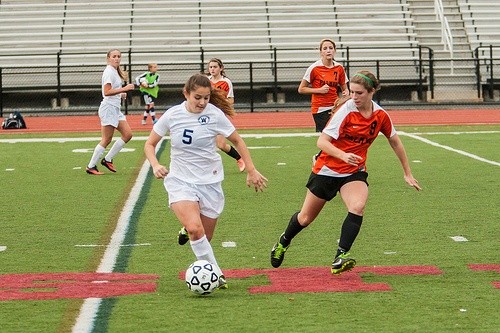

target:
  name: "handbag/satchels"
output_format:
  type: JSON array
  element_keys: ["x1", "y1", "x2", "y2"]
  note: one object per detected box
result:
[{"x1": 1, "y1": 112, "x2": 26, "y2": 129}]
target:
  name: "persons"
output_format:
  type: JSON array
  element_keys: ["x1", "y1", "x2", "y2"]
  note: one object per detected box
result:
[
  {"x1": 85, "y1": 48, "x2": 135, "y2": 175},
  {"x1": 298, "y1": 38, "x2": 350, "y2": 166},
  {"x1": 206, "y1": 58, "x2": 246, "y2": 172},
  {"x1": 142, "y1": 72, "x2": 269, "y2": 290},
  {"x1": 136, "y1": 63, "x2": 160, "y2": 125},
  {"x1": 270, "y1": 69, "x2": 423, "y2": 275}
]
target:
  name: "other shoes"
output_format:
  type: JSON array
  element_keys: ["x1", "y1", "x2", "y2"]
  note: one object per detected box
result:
[{"x1": 312, "y1": 154, "x2": 317, "y2": 170}]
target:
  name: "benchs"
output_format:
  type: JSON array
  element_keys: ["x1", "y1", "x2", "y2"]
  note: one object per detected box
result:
[{"x1": 0, "y1": 0, "x2": 500, "y2": 84}]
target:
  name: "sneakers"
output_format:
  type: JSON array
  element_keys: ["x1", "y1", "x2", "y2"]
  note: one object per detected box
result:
[
  {"x1": 101, "y1": 158, "x2": 116, "y2": 172},
  {"x1": 237, "y1": 156, "x2": 245, "y2": 173},
  {"x1": 177, "y1": 226, "x2": 189, "y2": 245},
  {"x1": 152, "y1": 120, "x2": 157, "y2": 124},
  {"x1": 330, "y1": 251, "x2": 356, "y2": 274},
  {"x1": 215, "y1": 276, "x2": 228, "y2": 290},
  {"x1": 86, "y1": 165, "x2": 104, "y2": 175},
  {"x1": 271, "y1": 232, "x2": 291, "y2": 268},
  {"x1": 142, "y1": 120, "x2": 146, "y2": 125}
]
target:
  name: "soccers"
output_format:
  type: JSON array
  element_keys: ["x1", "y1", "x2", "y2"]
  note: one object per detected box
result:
[{"x1": 185, "y1": 258, "x2": 223, "y2": 296}]
